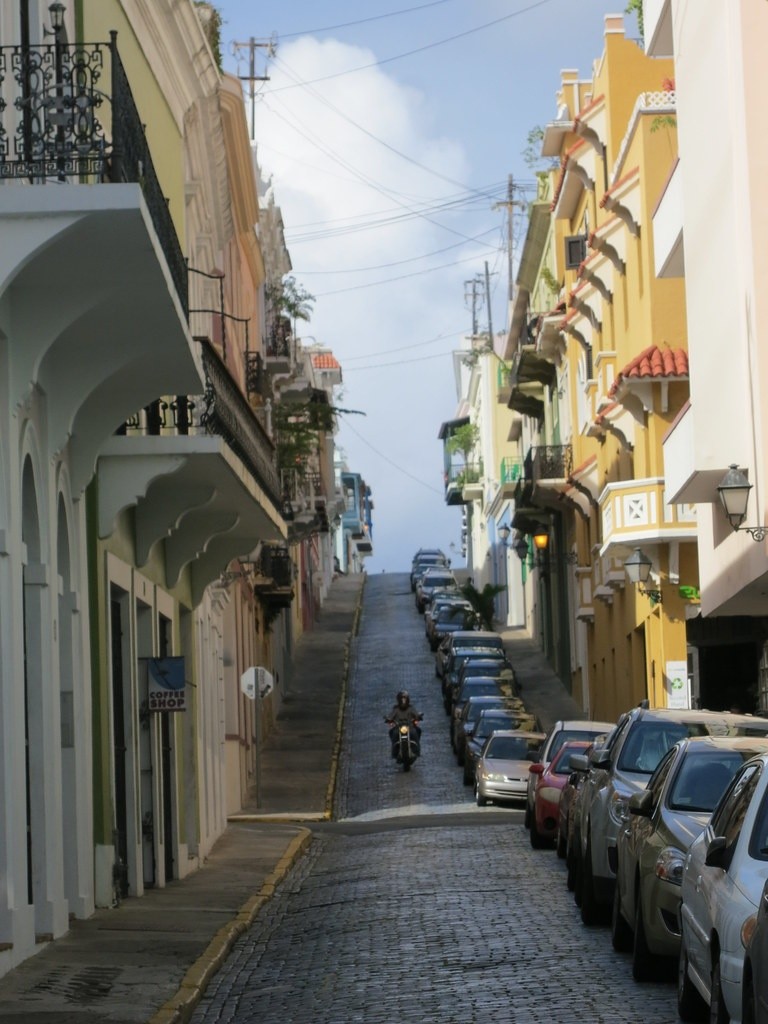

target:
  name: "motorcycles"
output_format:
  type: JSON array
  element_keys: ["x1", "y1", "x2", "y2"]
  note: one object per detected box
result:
[{"x1": 383, "y1": 711, "x2": 427, "y2": 771}]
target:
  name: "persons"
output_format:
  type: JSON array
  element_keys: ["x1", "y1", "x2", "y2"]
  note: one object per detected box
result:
[{"x1": 385, "y1": 691, "x2": 424, "y2": 759}]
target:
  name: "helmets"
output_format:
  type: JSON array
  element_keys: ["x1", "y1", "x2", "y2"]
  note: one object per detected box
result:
[{"x1": 397, "y1": 690, "x2": 409, "y2": 698}]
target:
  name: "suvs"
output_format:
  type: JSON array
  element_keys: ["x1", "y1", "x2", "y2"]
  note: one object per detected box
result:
[
  {"x1": 568, "y1": 700, "x2": 768, "y2": 924},
  {"x1": 419, "y1": 567, "x2": 455, "y2": 578},
  {"x1": 451, "y1": 696, "x2": 533, "y2": 758},
  {"x1": 414, "y1": 574, "x2": 460, "y2": 612},
  {"x1": 461, "y1": 708, "x2": 544, "y2": 785},
  {"x1": 411, "y1": 563, "x2": 452, "y2": 589},
  {"x1": 448, "y1": 676, "x2": 526, "y2": 724},
  {"x1": 420, "y1": 589, "x2": 466, "y2": 622},
  {"x1": 424, "y1": 596, "x2": 479, "y2": 633},
  {"x1": 610, "y1": 736, "x2": 767, "y2": 986},
  {"x1": 413, "y1": 547, "x2": 445, "y2": 556},
  {"x1": 447, "y1": 658, "x2": 521, "y2": 714},
  {"x1": 411, "y1": 557, "x2": 451, "y2": 568},
  {"x1": 525, "y1": 720, "x2": 619, "y2": 828},
  {"x1": 444, "y1": 646, "x2": 512, "y2": 692}
]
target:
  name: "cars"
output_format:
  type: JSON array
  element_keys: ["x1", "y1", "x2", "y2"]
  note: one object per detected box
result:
[
  {"x1": 435, "y1": 631, "x2": 505, "y2": 675},
  {"x1": 556, "y1": 734, "x2": 611, "y2": 857},
  {"x1": 742, "y1": 876, "x2": 768, "y2": 1024},
  {"x1": 428, "y1": 610, "x2": 485, "y2": 652},
  {"x1": 472, "y1": 729, "x2": 548, "y2": 807},
  {"x1": 528, "y1": 742, "x2": 595, "y2": 851},
  {"x1": 675, "y1": 754, "x2": 768, "y2": 1024}
]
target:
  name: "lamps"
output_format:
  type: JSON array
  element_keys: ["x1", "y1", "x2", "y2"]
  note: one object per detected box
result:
[
  {"x1": 499, "y1": 523, "x2": 513, "y2": 550},
  {"x1": 623, "y1": 549, "x2": 653, "y2": 586},
  {"x1": 515, "y1": 536, "x2": 530, "y2": 565},
  {"x1": 718, "y1": 464, "x2": 768, "y2": 545},
  {"x1": 531, "y1": 523, "x2": 549, "y2": 550}
]
